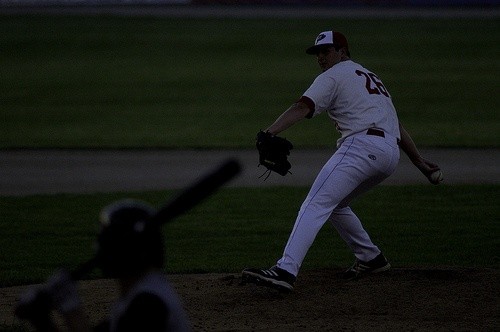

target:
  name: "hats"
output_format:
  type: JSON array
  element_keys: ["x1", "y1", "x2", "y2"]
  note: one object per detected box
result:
[{"x1": 305, "y1": 30, "x2": 347, "y2": 55}]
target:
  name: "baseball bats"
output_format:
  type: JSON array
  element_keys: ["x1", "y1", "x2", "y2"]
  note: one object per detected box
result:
[{"x1": 13, "y1": 158, "x2": 242, "y2": 321}]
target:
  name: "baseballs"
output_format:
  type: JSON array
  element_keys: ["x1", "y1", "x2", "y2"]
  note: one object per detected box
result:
[{"x1": 430, "y1": 170, "x2": 443, "y2": 184}]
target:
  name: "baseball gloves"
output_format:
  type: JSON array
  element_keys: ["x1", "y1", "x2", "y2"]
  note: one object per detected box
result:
[{"x1": 255, "y1": 130, "x2": 293, "y2": 176}]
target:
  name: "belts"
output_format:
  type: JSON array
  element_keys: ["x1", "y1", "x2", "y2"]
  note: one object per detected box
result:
[{"x1": 367, "y1": 128, "x2": 399, "y2": 143}]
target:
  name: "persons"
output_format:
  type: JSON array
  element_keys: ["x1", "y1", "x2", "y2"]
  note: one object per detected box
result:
[
  {"x1": 238, "y1": 28, "x2": 440, "y2": 292},
  {"x1": 15, "y1": 198, "x2": 193, "y2": 332}
]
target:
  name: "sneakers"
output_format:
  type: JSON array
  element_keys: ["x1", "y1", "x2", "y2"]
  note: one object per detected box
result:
[
  {"x1": 240, "y1": 265, "x2": 296, "y2": 294},
  {"x1": 331, "y1": 248, "x2": 391, "y2": 281}
]
